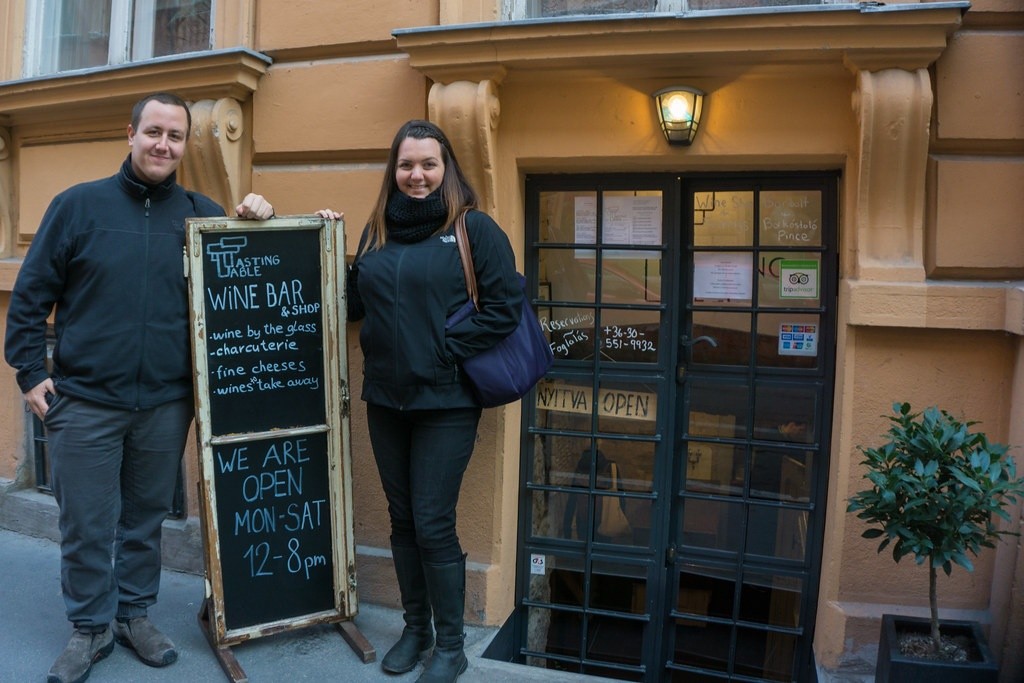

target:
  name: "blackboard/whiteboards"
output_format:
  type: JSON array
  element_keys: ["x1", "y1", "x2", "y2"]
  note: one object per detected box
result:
[{"x1": 185, "y1": 216, "x2": 359, "y2": 647}]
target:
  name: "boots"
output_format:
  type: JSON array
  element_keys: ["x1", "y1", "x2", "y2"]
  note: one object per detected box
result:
[
  {"x1": 416, "y1": 552, "x2": 469, "y2": 683},
  {"x1": 379, "y1": 537, "x2": 433, "y2": 674}
]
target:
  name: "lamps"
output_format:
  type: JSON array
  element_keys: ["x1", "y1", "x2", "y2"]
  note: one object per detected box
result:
[{"x1": 651, "y1": 84, "x2": 707, "y2": 146}]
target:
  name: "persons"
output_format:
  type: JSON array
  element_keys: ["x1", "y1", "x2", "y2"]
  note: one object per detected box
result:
[
  {"x1": 315, "y1": 119, "x2": 522, "y2": 683},
  {"x1": 5, "y1": 93, "x2": 274, "y2": 683},
  {"x1": 750, "y1": 413, "x2": 809, "y2": 554},
  {"x1": 563, "y1": 448, "x2": 620, "y2": 548}
]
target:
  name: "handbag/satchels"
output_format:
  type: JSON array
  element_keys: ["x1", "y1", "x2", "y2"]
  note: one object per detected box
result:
[
  {"x1": 597, "y1": 462, "x2": 633, "y2": 538},
  {"x1": 443, "y1": 211, "x2": 554, "y2": 409}
]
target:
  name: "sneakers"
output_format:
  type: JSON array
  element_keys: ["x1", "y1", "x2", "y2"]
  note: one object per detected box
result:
[
  {"x1": 111, "y1": 619, "x2": 177, "y2": 667},
  {"x1": 46, "y1": 626, "x2": 114, "y2": 683}
]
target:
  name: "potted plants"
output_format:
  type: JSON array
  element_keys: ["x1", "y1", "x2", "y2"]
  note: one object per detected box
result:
[{"x1": 845, "y1": 398, "x2": 1024, "y2": 683}]
